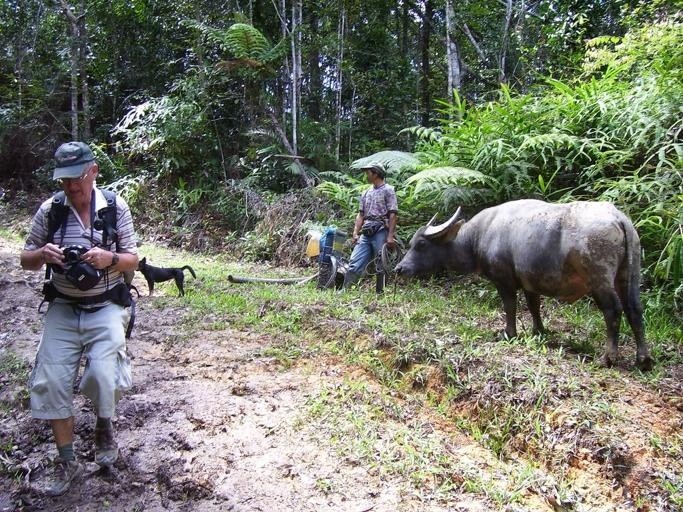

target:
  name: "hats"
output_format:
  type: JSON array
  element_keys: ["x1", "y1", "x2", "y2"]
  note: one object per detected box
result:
[
  {"x1": 359, "y1": 162, "x2": 386, "y2": 177},
  {"x1": 50, "y1": 141, "x2": 96, "y2": 183}
]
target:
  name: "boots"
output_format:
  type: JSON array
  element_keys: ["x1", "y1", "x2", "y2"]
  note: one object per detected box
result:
[
  {"x1": 375, "y1": 273, "x2": 385, "y2": 296},
  {"x1": 336, "y1": 272, "x2": 359, "y2": 295}
]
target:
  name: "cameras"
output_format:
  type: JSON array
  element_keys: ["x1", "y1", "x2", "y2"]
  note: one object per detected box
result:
[{"x1": 51, "y1": 244, "x2": 89, "y2": 275}]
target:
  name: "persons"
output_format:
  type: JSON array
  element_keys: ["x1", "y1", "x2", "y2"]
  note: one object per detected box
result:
[
  {"x1": 17, "y1": 139, "x2": 140, "y2": 496},
  {"x1": 338, "y1": 160, "x2": 398, "y2": 300}
]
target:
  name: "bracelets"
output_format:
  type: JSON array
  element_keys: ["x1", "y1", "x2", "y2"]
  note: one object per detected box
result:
[{"x1": 109, "y1": 251, "x2": 118, "y2": 268}]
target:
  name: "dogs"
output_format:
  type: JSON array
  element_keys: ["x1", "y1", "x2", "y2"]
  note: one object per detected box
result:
[{"x1": 134, "y1": 255, "x2": 198, "y2": 300}]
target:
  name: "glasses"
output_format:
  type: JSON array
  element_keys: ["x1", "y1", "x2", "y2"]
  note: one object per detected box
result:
[{"x1": 59, "y1": 168, "x2": 91, "y2": 184}]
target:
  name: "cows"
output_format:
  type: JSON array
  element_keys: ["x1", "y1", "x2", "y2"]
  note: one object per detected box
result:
[{"x1": 392, "y1": 198, "x2": 654, "y2": 375}]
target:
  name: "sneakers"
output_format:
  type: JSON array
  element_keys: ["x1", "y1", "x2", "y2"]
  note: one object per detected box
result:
[
  {"x1": 41, "y1": 458, "x2": 83, "y2": 497},
  {"x1": 91, "y1": 426, "x2": 120, "y2": 466}
]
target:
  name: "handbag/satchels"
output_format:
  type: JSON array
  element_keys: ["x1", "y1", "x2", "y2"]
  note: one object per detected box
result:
[
  {"x1": 360, "y1": 220, "x2": 384, "y2": 237},
  {"x1": 107, "y1": 281, "x2": 131, "y2": 307}
]
